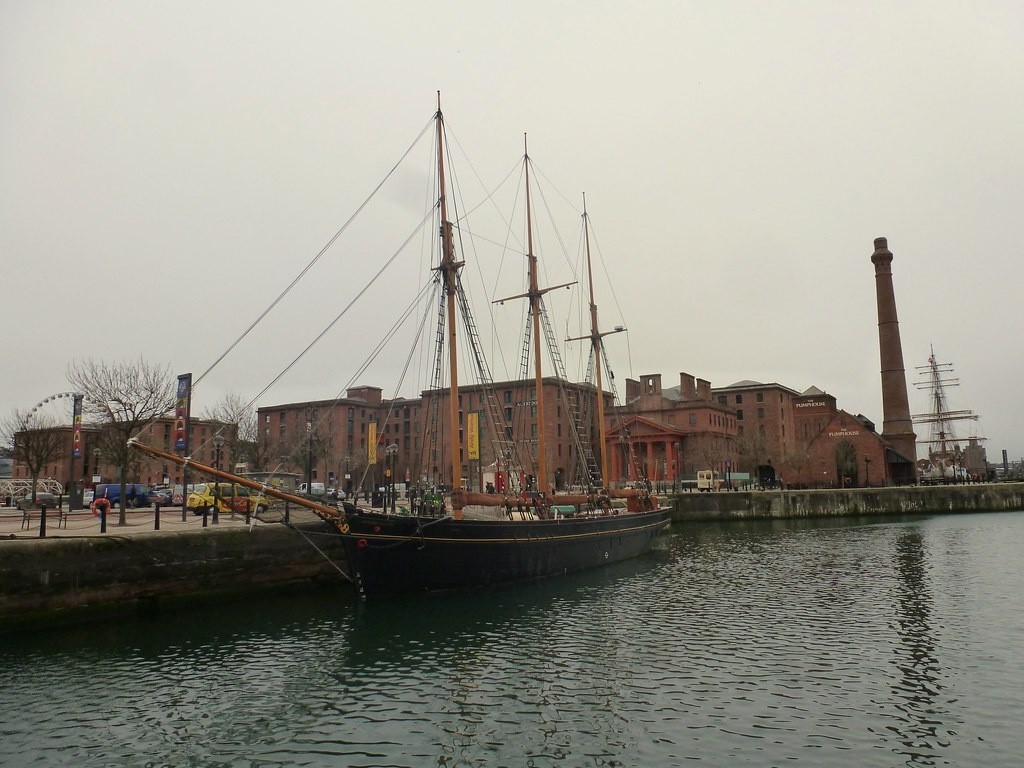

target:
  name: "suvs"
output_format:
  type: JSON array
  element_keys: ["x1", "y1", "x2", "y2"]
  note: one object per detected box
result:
[{"x1": 327, "y1": 488, "x2": 346, "y2": 501}]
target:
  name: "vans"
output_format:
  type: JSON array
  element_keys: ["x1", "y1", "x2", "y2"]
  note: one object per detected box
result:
[
  {"x1": 698, "y1": 471, "x2": 720, "y2": 492},
  {"x1": 295, "y1": 483, "x2": 324, "y2": 497},
  {"x1": 126, "y1": 90, "x2": 673, "y2": 603}
]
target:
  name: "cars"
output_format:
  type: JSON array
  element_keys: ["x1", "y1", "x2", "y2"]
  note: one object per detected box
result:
[
  {"x1": 83, "y1": 483, "x2": 268, "y2": 516},
  {"x1": 16, "y1": 492, "x2": 57, "y2": 510}
]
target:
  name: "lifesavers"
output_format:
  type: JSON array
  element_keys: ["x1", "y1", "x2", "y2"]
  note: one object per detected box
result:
[
  {"x1": 92, "y1": 498, "x2": 110, "y2": 517},
  {"x1": 358, "y1": 539, "x2": 367, "y2": 547}
]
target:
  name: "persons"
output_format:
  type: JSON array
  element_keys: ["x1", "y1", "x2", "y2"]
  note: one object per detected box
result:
[
  {"x1": 486, "y1": 482, "x2": 494, "y2": 494},
  {"x1": 468, "y1": 483, "x2": 472, "y2": 492},
  {"x1": 549, "y1": 485, "x2": 555, "y2": 495}
]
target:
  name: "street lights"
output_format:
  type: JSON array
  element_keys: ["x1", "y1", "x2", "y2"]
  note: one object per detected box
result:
[
  {"x1": 386, "y1": 444, "x2": 398, "y2": 514},
  {"x1": 344, "y1": 456, "x2": 350, "y2": 475},
  {"x1": 93, "y1": 448, "x2": 102, "y2": 474},
  {"x1": 212, "y1": 436, "x2": 224, "y2": 523}
]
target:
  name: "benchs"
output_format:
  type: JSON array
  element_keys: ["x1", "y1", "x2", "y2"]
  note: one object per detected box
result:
[{"x1": 22, "y1": 508, "x2": 67, "y2": 531}]
